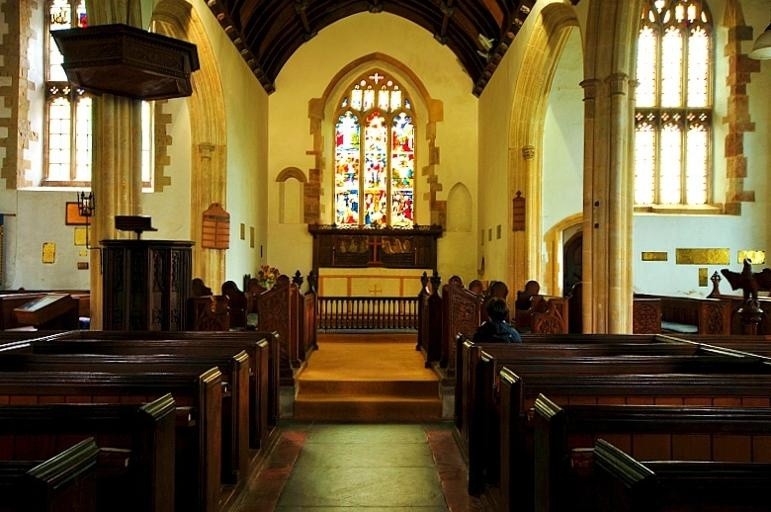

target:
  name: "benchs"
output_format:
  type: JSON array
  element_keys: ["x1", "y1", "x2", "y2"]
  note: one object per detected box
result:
[
  {"x1": 415, "y1": 267, "x2": 770, "y2": 511},
  {"x1": 0, "y1": 267, "x2": 319, "y2": 512}
]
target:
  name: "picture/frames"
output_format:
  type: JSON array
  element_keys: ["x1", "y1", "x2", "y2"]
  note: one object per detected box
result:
[{"x1": 64, "y1": 201, "x2": 91, "y2": 226}]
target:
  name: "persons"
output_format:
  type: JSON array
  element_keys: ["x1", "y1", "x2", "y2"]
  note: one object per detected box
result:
[{"x1": 470, "y1": 297, "x2": 524, "y2": 345}]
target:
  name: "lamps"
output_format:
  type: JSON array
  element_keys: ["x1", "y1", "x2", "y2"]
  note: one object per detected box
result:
[{"x1": 75, "y1": 191, "x2": 102, "y2": 274}]
